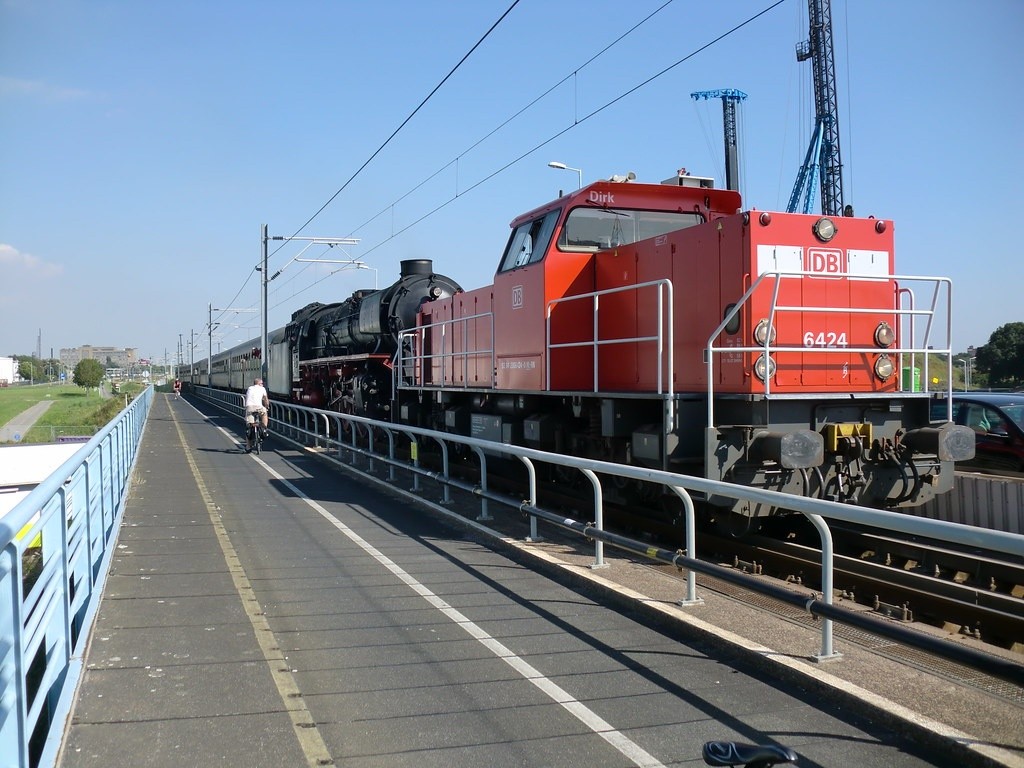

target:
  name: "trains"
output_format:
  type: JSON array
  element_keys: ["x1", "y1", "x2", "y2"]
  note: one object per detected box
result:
[{"x1": 173, "y1": 175, "x2": 977, "y2": 547}]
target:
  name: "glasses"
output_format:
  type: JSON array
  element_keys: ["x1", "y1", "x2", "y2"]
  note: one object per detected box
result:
[{"x1": 259, "y1": 382, "x2": 264, "y2": 385}]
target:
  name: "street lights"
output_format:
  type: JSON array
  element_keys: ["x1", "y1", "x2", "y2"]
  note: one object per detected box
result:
[
  {"x1": 357, "y1": 265, "x2": 378, "y2": 290},
  {"x1": 548, "y1": 161, "x2": 582, "y2": 190},
  {"x1": 234, "y1": 325, "x2": 262, "y2": 340}
]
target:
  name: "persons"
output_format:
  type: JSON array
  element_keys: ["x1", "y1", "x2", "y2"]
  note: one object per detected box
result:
[
  {"x1": 245, "y1": 378, "x2": 270, "y2": 454},
  {"x1": 172, "y1": 379, "x2": 181, "y2": 399}
]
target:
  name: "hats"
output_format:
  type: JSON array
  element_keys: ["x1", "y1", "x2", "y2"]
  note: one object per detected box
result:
[{"x1": 176, "y1": 378, "x2": 179, "y2": 381}]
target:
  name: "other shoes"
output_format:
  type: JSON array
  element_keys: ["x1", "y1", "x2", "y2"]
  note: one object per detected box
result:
[
  {"x1": 244, "y1": 445, "x2": 250, "y2": 451},
  {"x1": 174, "y1": 396, "x2": 176, "y2": 399},
  {"x1": 178, "y1": 392, "x2": 181, "y2": 396}
]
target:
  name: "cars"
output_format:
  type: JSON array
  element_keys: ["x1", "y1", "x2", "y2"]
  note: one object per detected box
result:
[{"x1": 931, "y1": 390, "x2": 1024, "y2": 472}]
